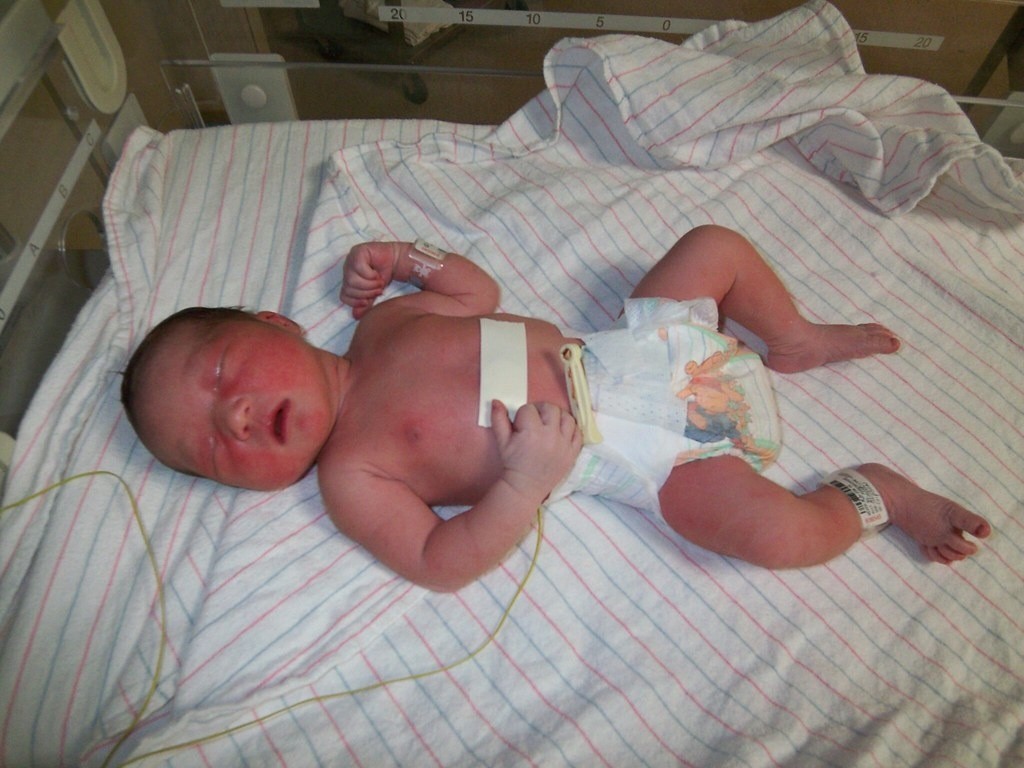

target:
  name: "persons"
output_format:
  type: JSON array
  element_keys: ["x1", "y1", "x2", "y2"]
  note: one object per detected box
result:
[{"x1": 121, "y1": 225, "x2": 992, "y2": 591}]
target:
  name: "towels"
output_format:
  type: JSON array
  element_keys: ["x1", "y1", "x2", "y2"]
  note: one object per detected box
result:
[{"x1": 2, "y1": 6, "x2": 1023, "y2": 766}]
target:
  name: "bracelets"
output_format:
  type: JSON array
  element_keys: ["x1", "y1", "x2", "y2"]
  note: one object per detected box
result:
[{"x1": 406, "y1": 238, "x2": 448, "y2": 289}]
